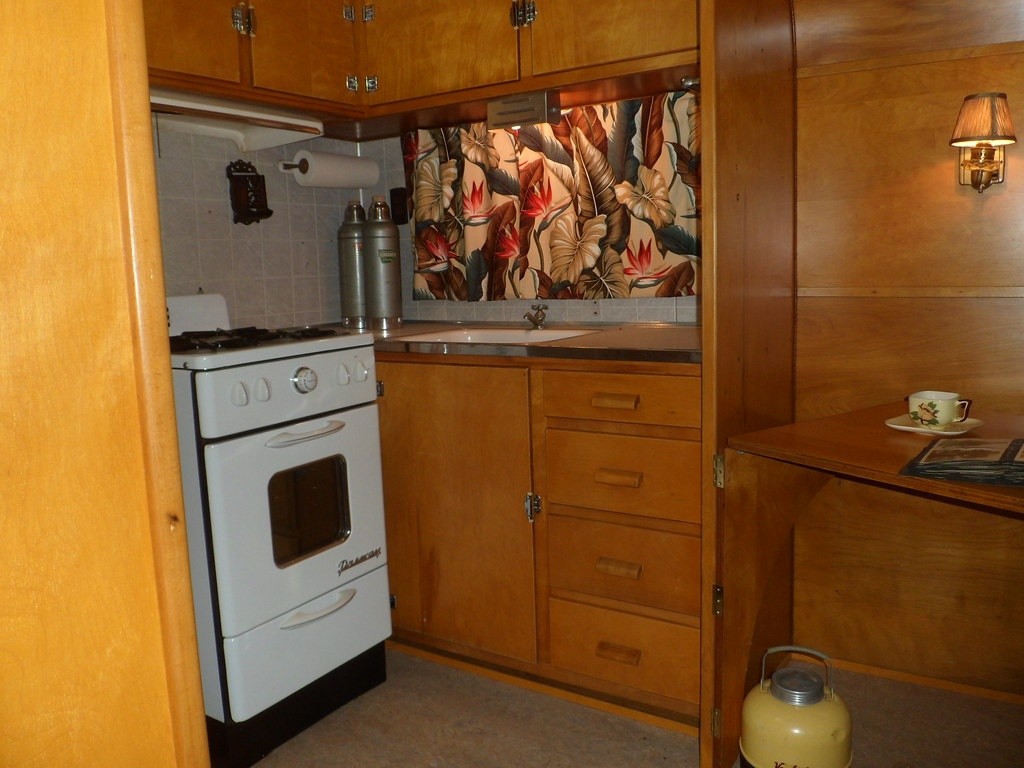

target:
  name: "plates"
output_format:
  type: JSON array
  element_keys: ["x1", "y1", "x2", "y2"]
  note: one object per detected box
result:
[{"x1": 885, "y1": 414, "x2": 985, "y2": 436}]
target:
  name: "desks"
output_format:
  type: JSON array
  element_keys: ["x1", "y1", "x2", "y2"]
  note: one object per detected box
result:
[{"x1": 717, "y1": 399, "x2": 1023, "y2": 768}]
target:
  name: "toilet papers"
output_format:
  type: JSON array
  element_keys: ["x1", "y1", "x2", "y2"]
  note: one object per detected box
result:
[{"x1": 292, "y1": 149, "x2": 380, "y2": 189}]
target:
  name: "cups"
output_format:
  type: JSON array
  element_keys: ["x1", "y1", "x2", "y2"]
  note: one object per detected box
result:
[{"x1": 908, "y1": 391, "x2": 968, "y2": 429}]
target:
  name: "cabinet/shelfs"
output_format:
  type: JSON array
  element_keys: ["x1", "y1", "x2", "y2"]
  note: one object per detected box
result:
[
  {"x1": 142, "y1": 1, "x2": 358, "y2": 142},
  {"x1": 354, "y1": 1, "x2": 698, "y2": 144},
  {"x1": 541, "y1": 360, "x2": 702, "y2": 734},
  {"x1": 300, "y1": 350, "x2": 530, "y2": 688}
]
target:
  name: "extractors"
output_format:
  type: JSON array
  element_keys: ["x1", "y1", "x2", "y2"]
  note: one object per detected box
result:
[{"x1": 147, "y1": 85, "x2": 325, "y2": 153}]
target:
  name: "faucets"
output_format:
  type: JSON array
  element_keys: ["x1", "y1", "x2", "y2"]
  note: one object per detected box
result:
[{"x1": 523, "y1": 304, "x2": 549, "y2": 330}]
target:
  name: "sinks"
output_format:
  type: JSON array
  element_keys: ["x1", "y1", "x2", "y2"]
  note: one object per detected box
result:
[{"x1": 384, "y1": 325, "x2": 606, "y2": 345}]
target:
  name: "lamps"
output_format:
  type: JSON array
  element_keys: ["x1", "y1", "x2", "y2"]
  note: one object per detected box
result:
[{"x1": 950, "y1": 92, "x2": 1017, "y2": 193}]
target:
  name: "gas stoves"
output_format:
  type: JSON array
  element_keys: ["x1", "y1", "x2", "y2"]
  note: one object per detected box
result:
[{"x1": 169, "y1": 322, "x2": 371, "y2": 361}]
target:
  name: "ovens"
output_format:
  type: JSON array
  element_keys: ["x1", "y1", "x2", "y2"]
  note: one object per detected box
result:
[{"x1": 170, "y1": 345, "x2": 391, "y2": 767}]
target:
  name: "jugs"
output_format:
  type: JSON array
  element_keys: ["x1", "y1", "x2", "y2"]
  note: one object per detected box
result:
[
  {"x1": 739, "y1": 647, "x2": 853, "y2": 768},
  {"x1": 337, "y1": 200, "x2": 367, "y2": 330},
  {"x1": 363, "y1": 195, "x2": 402, "y2": 329}
]
